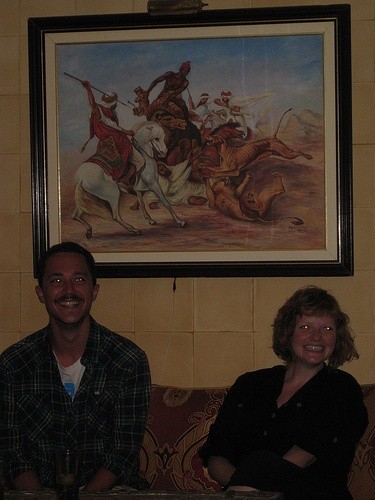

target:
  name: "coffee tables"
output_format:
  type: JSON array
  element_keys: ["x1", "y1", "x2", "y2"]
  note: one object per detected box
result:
[{"x1": 0, "y1": 490, "x2": 284, "y2": 500}]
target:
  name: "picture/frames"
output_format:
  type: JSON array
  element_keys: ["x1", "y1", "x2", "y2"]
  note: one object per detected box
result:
[{"x1": 28, "y1": 4, "x2": 354, "y2": 279}]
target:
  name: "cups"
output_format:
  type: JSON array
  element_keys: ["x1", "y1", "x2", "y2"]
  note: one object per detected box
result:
[{"x1": 54, "y1": 448, "x2": 80, "y2": 500}]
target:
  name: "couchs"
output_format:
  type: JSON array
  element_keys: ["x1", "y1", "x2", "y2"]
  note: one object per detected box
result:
[{"x1": 140, "y1": 384, "x2": 375, "y2": 500}]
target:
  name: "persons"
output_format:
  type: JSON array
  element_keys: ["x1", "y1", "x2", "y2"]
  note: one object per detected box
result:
[
  {"x1": 1, "y1": 242, "x2": 153, "y2": 495},
  {"x1": 190, "y1": 283, "x2": 370, "y2": 500}
]
